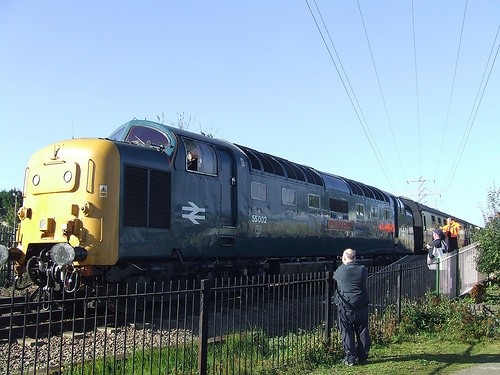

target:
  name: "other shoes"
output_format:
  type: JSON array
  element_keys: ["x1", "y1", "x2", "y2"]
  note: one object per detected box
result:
[{"x1": 342, "y1": 359, "x2": 367, "y2": 366}]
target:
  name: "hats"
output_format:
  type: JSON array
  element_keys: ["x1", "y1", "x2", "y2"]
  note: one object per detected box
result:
[{"x1": 432, "y1": 228, "x2": 445, "y2": 236}]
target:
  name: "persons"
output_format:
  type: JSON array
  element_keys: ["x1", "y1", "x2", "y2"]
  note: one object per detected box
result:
[
  {"x1": 438, "y1": 218, "x2": 461, "y2": 252},
  {"x1": 334, "y1": 248, "x2": 371, "y2": 366},
  {"x1": 187, "y1": 151, "x2": 198, "y2": 171},
  {"x1": 426, "y1": 230, "x2": 449, "y2": 291}
]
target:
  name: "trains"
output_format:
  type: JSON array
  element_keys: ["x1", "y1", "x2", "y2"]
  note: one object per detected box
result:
[{"x1": 0, "y1": 120, "x2": 485, "y2": 318}]
